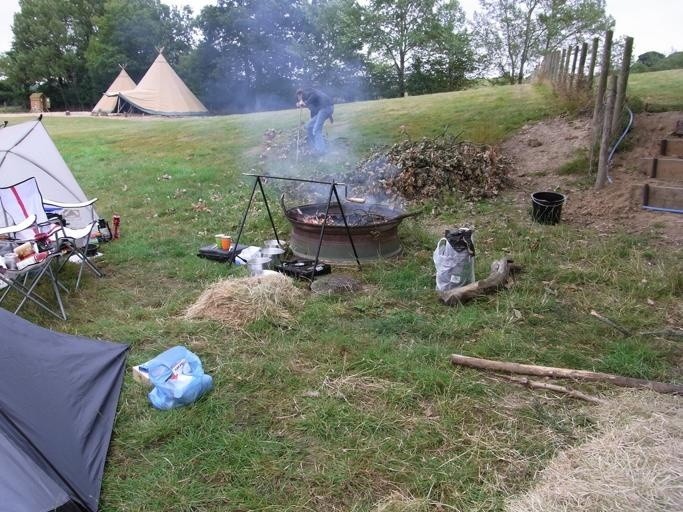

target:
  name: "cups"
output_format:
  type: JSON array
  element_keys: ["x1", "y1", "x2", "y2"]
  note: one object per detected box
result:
[
  {"x1": 4, "y1": 252, "x2": 18, "y2": 270},
  {"x1": 214, "y1": 233, "x2": 231, "y2": 251}
]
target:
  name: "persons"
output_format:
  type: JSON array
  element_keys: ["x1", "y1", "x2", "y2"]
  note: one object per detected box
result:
[{"x1": 295, "y1": 86, "x2": 334, "y2": 154}]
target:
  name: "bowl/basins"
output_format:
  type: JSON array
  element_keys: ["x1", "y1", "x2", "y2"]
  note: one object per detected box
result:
[
  {"x1": 253, "y1": 239, "x2": 286, "y2": 263},
  {"x1": 82, "y1": 243, "x2": 98, "y2": 257}
]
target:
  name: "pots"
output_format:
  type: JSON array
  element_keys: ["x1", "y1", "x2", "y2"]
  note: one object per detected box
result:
[
  {"x1": 279, "y1": 193, "x2": 424, "y2": 234},
  {"x1": 232, "y1": 254, "x2": 272, "y2": 275}
]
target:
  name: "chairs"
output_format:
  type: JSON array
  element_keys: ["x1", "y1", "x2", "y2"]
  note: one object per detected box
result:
[{"x1": 0, "y1": 176, "x2": 103, "y2": 321}]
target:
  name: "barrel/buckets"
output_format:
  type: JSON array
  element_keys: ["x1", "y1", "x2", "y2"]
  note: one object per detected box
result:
[
  {"x1": 246, "y1": 240, "x2": 287, "y2": 273},
  {"x1": 530, "y1": 190, "x2": 566, "y2": 225},
  {"x1": 96, "y1": 218, "x2": 113, "y2": 242}
]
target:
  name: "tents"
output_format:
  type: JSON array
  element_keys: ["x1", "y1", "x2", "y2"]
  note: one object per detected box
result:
[
  {"x1": 103, "y1": 47, "x2": 210, "y2": 118},
  {"x1": 91, "y1": 63, "x2": 138, "y2": 115},
  {"x1": 0, "y1": 306, "x2": 131, "y2": 512},
  {"x1": 0, "y1": 114, "x2": 101, "y2": 288}
]
